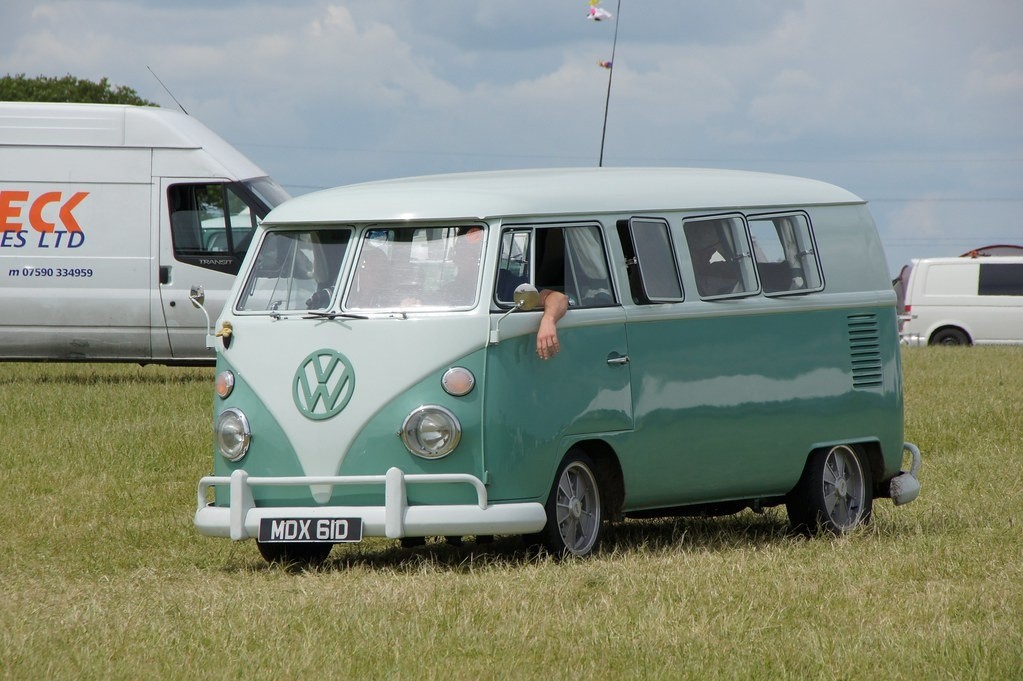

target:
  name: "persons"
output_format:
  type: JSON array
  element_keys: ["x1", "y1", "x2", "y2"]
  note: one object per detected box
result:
[{"x1": 400, "y1": 225, "x2": 570, "y2": 360}]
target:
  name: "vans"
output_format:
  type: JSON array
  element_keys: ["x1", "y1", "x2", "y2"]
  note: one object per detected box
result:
[
  {"x1": 892, "y1": 249, "x2": 1023, "y2": 348},
  {"x1": 0, "y1": 101, "x2": 330, "y2": 367},
  {"x1": 193, "y1": 165, "x2": 924, "y2": 577}
]
